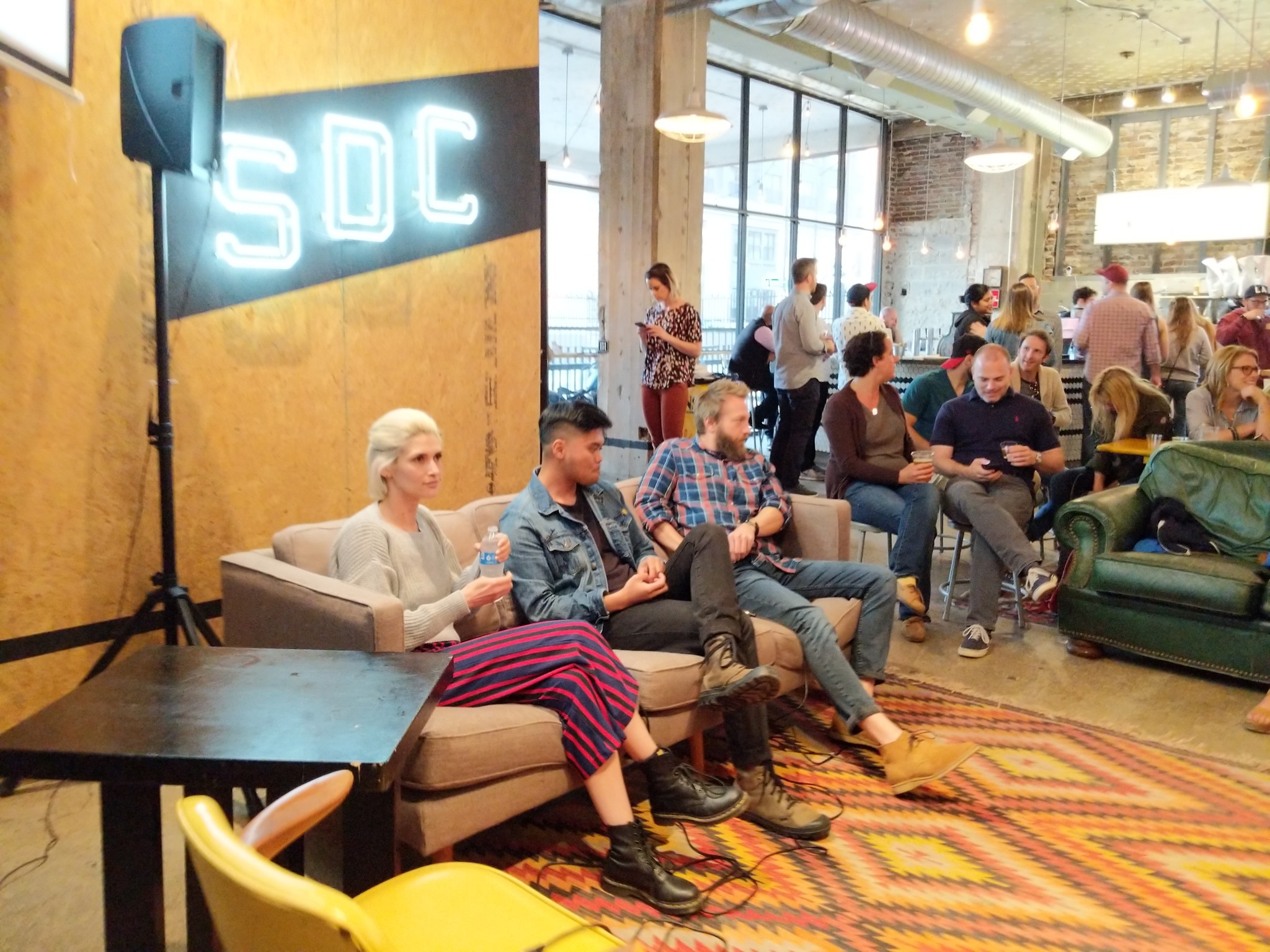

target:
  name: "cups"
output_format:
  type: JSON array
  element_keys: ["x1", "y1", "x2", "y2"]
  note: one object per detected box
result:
[
  {"x1": 1146, "y1": 434, "x2": 1162, "y2": 450},
  {"x1": 1000, "y1": 441, "x2": 1018, "y2": 459},
  {"x1": 911, "y1": 450, "x2": 935, "y2": 482},
  {"x1": 1200, "y1": 427, "x2": 1220, "y2": 441},
  {"x1": 1171, "y1": 436, "x2": 1188, "y2": 440}
]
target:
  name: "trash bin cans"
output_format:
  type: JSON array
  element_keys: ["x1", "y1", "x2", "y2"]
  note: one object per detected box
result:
[{"x1": 682, "y1": 373, "x2": 733, "y2": 439}]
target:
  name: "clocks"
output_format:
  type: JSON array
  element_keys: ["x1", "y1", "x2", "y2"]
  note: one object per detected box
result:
[{"x1": 983, "y1": 268, "x2": 1003, "y2": 288}]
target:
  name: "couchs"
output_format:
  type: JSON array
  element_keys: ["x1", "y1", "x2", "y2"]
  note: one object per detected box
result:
[{"x1": 1054, "y1": 440, "x2": 1270, "y2": 691}]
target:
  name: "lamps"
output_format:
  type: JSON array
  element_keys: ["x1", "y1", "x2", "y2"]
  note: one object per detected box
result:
[
  {"x1": 654, "y1": 7, "x2": 729, "y2": 144},
  {"x1": 1121, "y1": 12, "x2": 1149, "y2": 109},
  {"x1": 963, "y1": 118, "x2": 1034, "y2": 172},
  {"x1": 1235, "y1": 0, "x2": 1257, "y2": 117}
]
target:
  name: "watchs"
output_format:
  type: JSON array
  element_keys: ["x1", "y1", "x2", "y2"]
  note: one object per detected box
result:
[{"x1": 1033, "y1": 451, "x2": 1042, "y2": 467}]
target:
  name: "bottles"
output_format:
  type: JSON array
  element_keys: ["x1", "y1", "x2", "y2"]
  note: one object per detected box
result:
[{"x1": 480, "y1": 526, "x2": 504, "y2": 603}]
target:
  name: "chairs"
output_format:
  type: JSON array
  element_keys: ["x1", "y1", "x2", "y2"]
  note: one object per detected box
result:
[{"x1": 175, "y1": 767, "x2": 632, "y2": 952}]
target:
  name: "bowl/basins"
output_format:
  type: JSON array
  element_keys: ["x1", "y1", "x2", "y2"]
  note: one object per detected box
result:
[{"x1": 892, "y1": 345, "x2": 905, "y2": 359}]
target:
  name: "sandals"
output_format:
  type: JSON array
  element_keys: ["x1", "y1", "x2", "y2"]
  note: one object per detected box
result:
[{"x1": 1244, "y1": 688, "x2": 1270, "y2": 733}]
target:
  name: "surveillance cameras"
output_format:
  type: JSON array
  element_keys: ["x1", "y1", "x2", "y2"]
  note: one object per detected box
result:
[{"x1": 1066, "y1": 267, "x2": 1072, "y2": 276}]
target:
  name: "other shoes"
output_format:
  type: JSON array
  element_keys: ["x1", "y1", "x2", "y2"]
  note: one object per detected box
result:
[
  {"x1": 765, "y1": 429, "x2": 774, "y2": 450},
  {"x1": 748, "y1": 418, "x2": 769, "y2": 430}
]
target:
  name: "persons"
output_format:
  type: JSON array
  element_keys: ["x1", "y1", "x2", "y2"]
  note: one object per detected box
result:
[
  {"x1": 727, "y1": 304, "x2": 778, "y2": 440},
  {"x1": 496, "y1": 398, "x2": 831, "y2": 839},
  {"x1": 822, "y1": 330, "x2": 946, "y2": 640},
  {"x1": 927, "y1": 340, "x2": 1069, "y2": 657},
  {"x1": 825, "y1": 263, "x2": 1270, "y2": 595},
  {"x1": 631, "y1": 376, "x2": 983, "y2": 797},
  {"x1": 329, "y1": 405, "x2": 753, "y2": 915},
  {"x1": 797, "y1": 283, "x2": 835, "y2": 481},
  {"x1": 636, "y1": 262, "x2": 703, "y2": 457},
  {"x1": 1246, "y1": 690, "x2": 1269, "y2": 733},
  {"x1": 768, "y1": 257, "x2": 837, "y2": 498}
]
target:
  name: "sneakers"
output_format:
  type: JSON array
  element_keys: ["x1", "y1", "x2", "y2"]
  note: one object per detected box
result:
[
  {"x1": 644, "y1": 762, "x2": 748, "y2": 827},
  {"x1": 958, "y1": 624, "x2": 992, "y2": 658},
  {"x1": 602, "y1": 818, "x2": 702, "y2": 915},
  {"x1": 878, "y1": 731, "x2": 979, "y2": 797},
  {"x1": 1022, "y1": 566, "x2": 1058, "y2": 601},
  {"x1": 895, "y1": 581, "x2": 926, "y2": 615},
  {"x1": 829, "y1": 712, "x2": 882, "y2": 752},
  {"x1": 800, "y1": 464, "x2": 826, "y2": 481},
  {"x1": 785, "y1": 483, "x2": 818, "y2": 497},
  {"x1": 901, "y1": 616, "x2": 925, "y2": 642}
]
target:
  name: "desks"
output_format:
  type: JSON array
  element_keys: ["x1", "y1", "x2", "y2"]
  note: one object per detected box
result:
[
  {"x1": 2, "y1": 644, "x2": 456, "y2": 951},
  {"x1": 1096, "y1": 439, "x2": 1168, "y2": 463}
]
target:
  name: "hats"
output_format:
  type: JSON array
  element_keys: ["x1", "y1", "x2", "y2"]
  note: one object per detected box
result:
[
  {"x1": 1095, "y1": 264, "x2": 1128, "y2": 284},
  {"x1": 846, "y1": 283, "x2": 877, "y2": 302},
  {"x1": 940, "y1": 334, "x2": 988, "y2": 370},
  {"x1": 1244, "y1": 285, "x2": 1270, "y2": 299}
]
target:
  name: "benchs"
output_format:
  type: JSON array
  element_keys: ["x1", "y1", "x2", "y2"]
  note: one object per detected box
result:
[{"x1": 229, "y1": 475, "x2": 867, "y2": 866}]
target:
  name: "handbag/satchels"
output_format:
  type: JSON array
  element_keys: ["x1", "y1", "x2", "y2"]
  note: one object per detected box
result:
[{"x1": 1162, "y1": 378, "x2": 1171, "y2": 394}]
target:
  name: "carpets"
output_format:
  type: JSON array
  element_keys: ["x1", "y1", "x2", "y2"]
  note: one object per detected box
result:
[{"x1": 339, "y1": 672, "x2": 1270, "y2": 952}]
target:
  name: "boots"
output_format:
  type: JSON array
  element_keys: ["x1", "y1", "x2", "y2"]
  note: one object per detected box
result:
[
  {"x1": 737, "y1": 760, "x2": 831, "y2": 840},
  {"x1": 698, "y1": 634, "x2": 781, "y2": 711}
]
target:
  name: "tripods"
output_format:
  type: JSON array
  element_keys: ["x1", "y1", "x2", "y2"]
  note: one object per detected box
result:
[{"x1": 80, "y1": 165, "x2": 264, "y2": 822}]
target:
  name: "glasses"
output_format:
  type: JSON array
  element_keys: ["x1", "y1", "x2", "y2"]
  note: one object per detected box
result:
[
  {"x1": 1247, "y1": 299, "x2": 1270, "y2": 308},
  {"x1": 1230, "y1": 366, "x2": 1262, "y2": 376}
]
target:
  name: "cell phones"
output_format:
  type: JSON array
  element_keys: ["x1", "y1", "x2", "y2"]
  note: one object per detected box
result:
[
  {"x1": 986, "y1": 464, "x2": 1003, "y2": 472},
  {"x1": 635, "y1": 322, "x2": 646, "y2": 328}
]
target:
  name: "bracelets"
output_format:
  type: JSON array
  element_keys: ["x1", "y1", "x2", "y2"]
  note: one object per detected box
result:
[{"x1": 1228, "y1": 426, "x2": 1239, "y2": 441}]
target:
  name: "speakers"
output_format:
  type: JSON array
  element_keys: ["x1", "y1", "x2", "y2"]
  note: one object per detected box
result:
[{"x1": 119, "y1": 18, "x2": 226, "y2": 181}]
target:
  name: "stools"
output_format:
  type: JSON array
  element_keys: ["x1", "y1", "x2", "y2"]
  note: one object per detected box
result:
[
  {"x1": 748, "y1": 387, "x2": 766, "y2": 455},
  {"x1": 851, "y1": 522, "x2": 892, "y2": 568},
  {"x1": 938, "y1": 519, "x2": 1026, "y2": 628}
]
